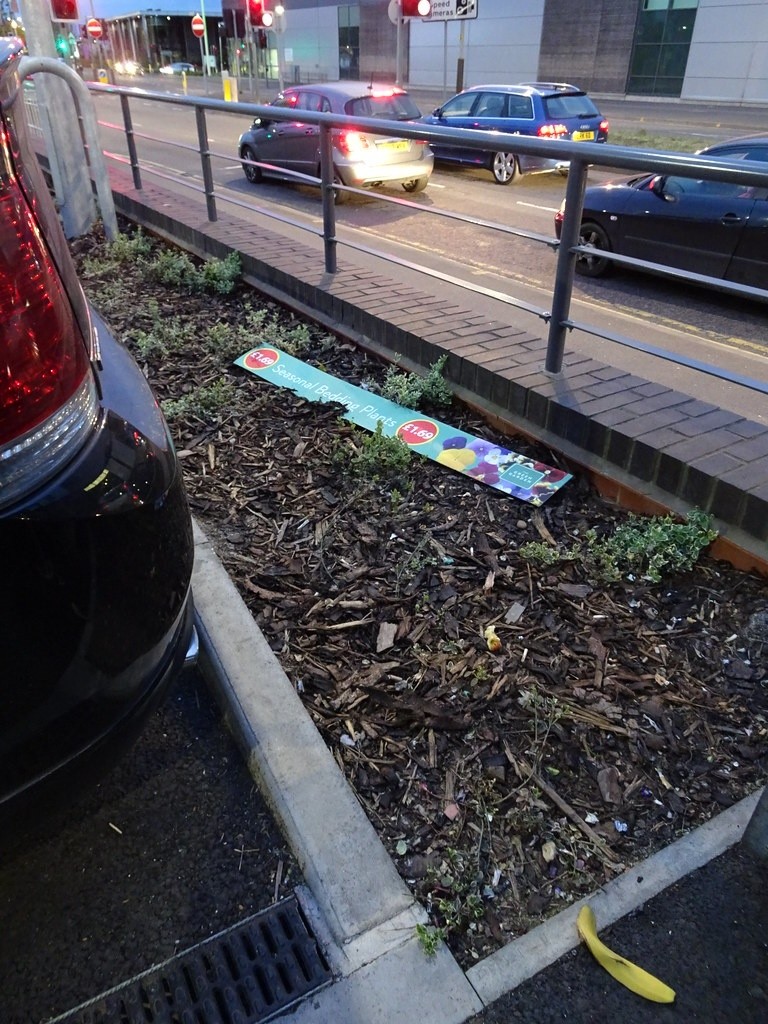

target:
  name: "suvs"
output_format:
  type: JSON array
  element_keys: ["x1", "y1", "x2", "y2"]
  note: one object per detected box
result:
[{"x1": 410, "y1": 81, "x2": 608, "y2": 185}]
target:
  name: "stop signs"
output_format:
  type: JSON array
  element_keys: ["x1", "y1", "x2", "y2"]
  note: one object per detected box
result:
[
  {"x1": 191, "y1": 17, "x2": 204, "y2": 37},
  {"x1": 86, "y1": 19, "x2": 102, "y2": 38}
]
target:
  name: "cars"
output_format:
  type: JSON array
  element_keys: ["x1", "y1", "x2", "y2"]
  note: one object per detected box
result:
[
  {"x1": 0, "y1": 0, "x2": 202, "y2": 812},
  {"x1": 115, "y1": 61, "x2": 145, "y2": 77},
  {"x1": 554, "y1": 132, "x2": 768, "y2": 303},
  {"x1": 159, "y1": 62, "x2": 195, "y2": 74},
  {"x1": 238, "y1": 81, "x2": 435, "y2": 205}
]
